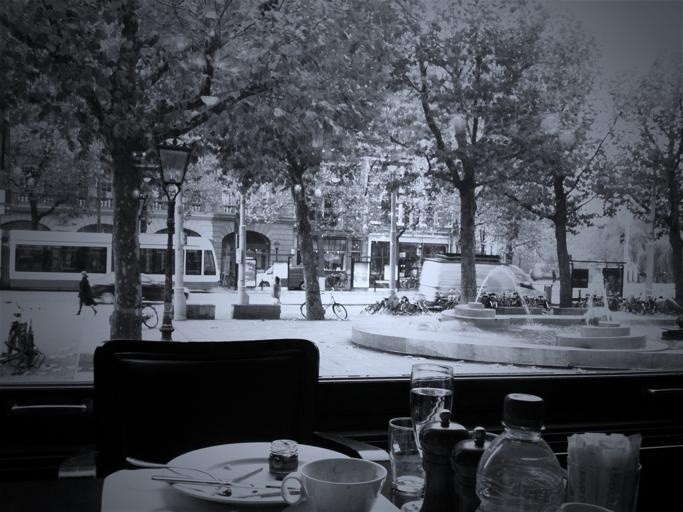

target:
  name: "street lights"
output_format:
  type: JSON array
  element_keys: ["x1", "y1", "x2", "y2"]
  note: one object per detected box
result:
[
  {"x1": 272, "y1": 239, "x2": 280, "y2": 261},
  {"x1": 147, "y1": 130, "x2": 195, "y2": 341}
]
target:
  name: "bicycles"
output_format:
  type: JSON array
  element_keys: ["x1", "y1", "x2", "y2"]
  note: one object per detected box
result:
[
  {"x1": 107, "y1": 299, "x2": 160, "y2": 334},
  {"x1": 300, "y1": 290, "x2": 351, "y2": 322},
  {"x1": 324, "y1": 271, "x2": 421, "y2": 290}
]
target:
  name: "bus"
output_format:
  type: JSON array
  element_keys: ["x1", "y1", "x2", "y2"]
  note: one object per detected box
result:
[{"x1": 3, "y1": 227, "x2": 223, "y2": 294}]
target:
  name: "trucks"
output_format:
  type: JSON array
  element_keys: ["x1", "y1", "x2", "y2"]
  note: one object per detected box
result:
[
  {"x1": 254, "y1": 261, "x2": 324, "y2": 292},
  {"x1": 419, "y1": 256, "x2": 538, "y2": 306}
]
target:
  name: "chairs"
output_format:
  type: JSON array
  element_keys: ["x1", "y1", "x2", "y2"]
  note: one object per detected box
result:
[{"x1": 58, "y1": 339, "x2": 394, "y2": 511}]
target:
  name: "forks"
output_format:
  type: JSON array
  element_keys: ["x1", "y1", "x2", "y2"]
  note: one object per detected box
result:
[{"x1": 126, "y1": 457, "x2": 264, "y2": 485}]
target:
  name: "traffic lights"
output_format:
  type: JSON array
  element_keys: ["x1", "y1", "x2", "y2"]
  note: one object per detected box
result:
[{"x1": 620, "y1": 232, "x2": 626, "y2": 245}]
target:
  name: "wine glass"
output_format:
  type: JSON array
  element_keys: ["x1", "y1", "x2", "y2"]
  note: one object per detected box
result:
[{"x1": 401, "y1": 364, "x2": 453, "y2": 512}]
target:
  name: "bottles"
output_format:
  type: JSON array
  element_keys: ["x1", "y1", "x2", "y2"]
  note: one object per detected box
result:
[
  {"x1": 420, "y1": 392, "x2": 563, "y2": 512},
  {"x1": 268, "y1": 439, "x2": 298, "y2": 480}
]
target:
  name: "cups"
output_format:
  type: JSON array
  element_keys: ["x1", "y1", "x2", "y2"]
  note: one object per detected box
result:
[
  {"x1": 389, "y1": 417, "x2": 426, "y2": 500},
  {"x1": 163, "y1": 442, "x2": 352, "y2": 505},
  {"x1": 280, "y1": 458, "x2": 389, "y2": 511},
  {"x1": 567, "y1": 457, "x2": 642, "y2": 511}
]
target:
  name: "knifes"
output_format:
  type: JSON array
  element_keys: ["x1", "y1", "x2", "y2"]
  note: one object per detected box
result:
[{"x1": 152, "y1": 476, "x2": 266, "y2": 491}]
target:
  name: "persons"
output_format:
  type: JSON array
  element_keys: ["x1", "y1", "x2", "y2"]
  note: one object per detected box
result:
[
  {"x1": 76, "y1": 271, "x2": 98, "y2": 315},
  {"x1": 273, "y1": 276, "x2": 281, "y2": 303}
]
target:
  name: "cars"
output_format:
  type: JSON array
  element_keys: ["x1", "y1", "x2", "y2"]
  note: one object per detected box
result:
[{"x1": 90, "y1": 270, "x2": 194, "y2": 304}]
